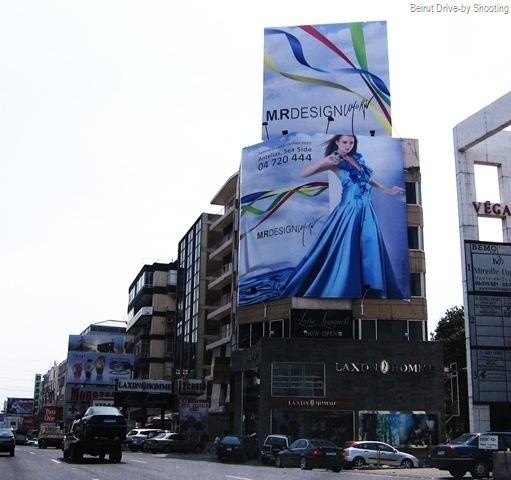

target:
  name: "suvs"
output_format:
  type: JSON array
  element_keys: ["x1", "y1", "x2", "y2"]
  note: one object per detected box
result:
[
  {"x1": 124, "y1": 428, "x2": 165, "y2": 452},
  {"x1": 260, "y1": 432, "x2": 292, "y2": 465}
]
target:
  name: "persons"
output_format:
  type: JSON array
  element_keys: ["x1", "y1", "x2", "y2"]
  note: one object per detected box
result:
[{"x1": 277, "y1": 135, "x2": 407, "y2": 299}]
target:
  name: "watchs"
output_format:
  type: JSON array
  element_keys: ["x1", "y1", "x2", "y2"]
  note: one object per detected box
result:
[
  {"x1": 95, "y1": 355, "x2": 105, "y2": 374},
  {"x1": 72, "y1": 361, "x2": 83, "y2": 378},
  {"x1": 109, "y1": 361, "x2": 129, "y2": 372},
  {"x1": 84, "y1": 359, "x2": 95, "y2": 377}
]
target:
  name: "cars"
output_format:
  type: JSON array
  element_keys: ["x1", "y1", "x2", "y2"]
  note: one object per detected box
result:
[
  {"x1": 341, "y1": 440, "x2": 423, "y2": 469},
  {"x1": 214, "y1": 434, "x2": 258, "y2": 464},
  {"x1": 426, "y1": 431, "x2": 510, "y2": 478},
  {"x1": 143, "y1": 432, "x2": 205, "y2": 455},
  {"x1": 26, "y1": 437, "x2": 38, "y2": 447},
  {"x1": 275, "y1": 438, "x2": 346, "y2": 473},
  {"x1": 136, "y1": 431, "x2": 162, "y2": 452},
  {"x1": 72, "y1": 406, "x2": 127, "y2": 443},
  {"x1": 0, "y1": 428, "x2": 15, "y2": 457}
]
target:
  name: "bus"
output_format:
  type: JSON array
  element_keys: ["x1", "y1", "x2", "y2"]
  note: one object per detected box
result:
[{"x1": 11, "y1": 429, "x2": 27, "y2": 445}]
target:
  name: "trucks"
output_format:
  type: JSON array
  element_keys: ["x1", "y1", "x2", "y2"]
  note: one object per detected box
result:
[
  {"x1": 37, "y1": 423, "x2": 66, "y2": 450},
  {"x1": 60, "y1": 419, "x2": 122, "y2": 464}
]
